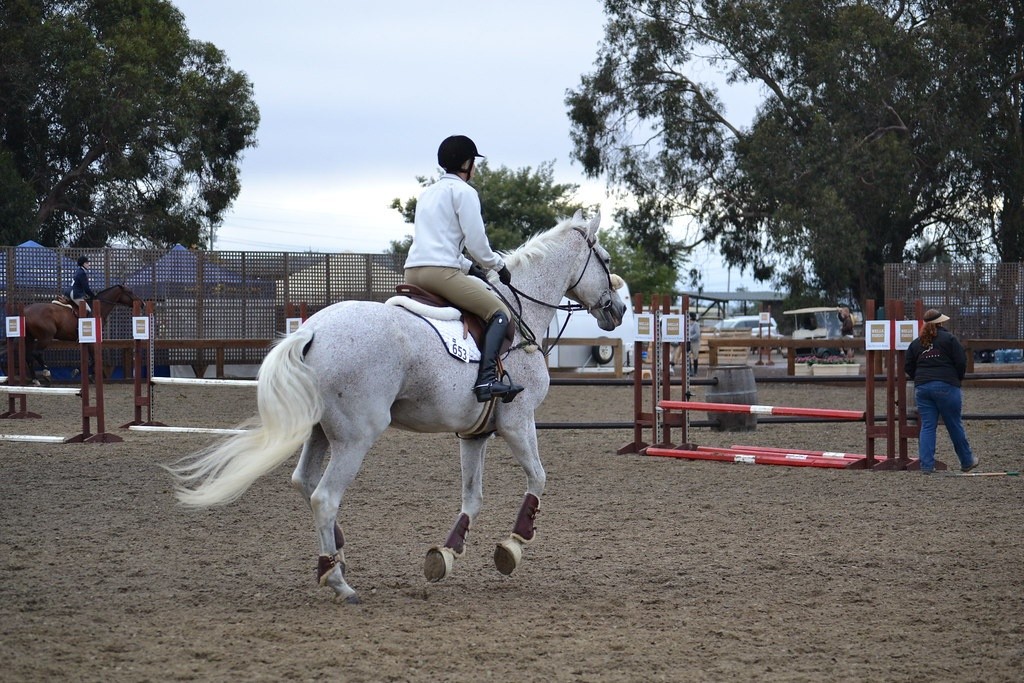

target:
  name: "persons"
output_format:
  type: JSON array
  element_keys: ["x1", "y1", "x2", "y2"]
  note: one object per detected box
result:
[
  {"x1": 689, "y1": 312, "x2": 700, "y2": 376},
  {"x1": 837, "y1": 306, "x2": 854, "y2": 362},
  {"x1": 404, "y1": 134, "x2": 524, "y2": 401},
  {"x1": 905, "y1": 309, "x2": 978, "y2": 474},
  {"x1": 65, "y1": 256, "x2": 96, "y2": 317}
]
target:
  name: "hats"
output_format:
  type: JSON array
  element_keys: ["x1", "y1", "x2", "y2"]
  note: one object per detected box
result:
[{"x1": 924, "y1": 309, "x2": 950, "y2": 324}]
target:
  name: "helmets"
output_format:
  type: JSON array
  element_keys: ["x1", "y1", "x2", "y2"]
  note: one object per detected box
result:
[
  {"x1": 77, "y1": 257, "x2": 91, "y2": 267},
  {"x1": 438, "y1": 135, "x2": 484, "y2": 171}
]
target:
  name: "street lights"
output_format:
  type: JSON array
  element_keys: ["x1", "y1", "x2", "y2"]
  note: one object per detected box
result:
[{"x1": 899, "y1": 266, "x2": 911, "y2": 320}]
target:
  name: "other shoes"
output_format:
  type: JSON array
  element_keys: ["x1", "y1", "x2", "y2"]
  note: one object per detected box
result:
[
  {"x1": 962, "y1": 458, "x2": 978, "y2": 472},
  {"x1": 923, "y1": 467, "x2": 936, "y2": 475}
]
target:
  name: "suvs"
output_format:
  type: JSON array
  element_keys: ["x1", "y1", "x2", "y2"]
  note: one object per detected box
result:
[{"x1": 711, "y1": 314, "x2": 779, "y2": 354}]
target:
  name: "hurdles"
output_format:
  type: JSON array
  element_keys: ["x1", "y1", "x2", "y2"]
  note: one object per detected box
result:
[
  {"x1": 535, "y1": 291, "x2": 1024, "y2": 473},
  {"x1": 0, "y1": 299, "x2": 309, "y2": 446}
]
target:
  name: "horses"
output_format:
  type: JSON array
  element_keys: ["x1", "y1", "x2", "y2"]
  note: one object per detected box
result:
[
  {"x1": 156, "y1": 208, "x2": 627, "y2": 606},
  {"x1": 0, "y1": 282, "x2": 145, "y2": 386}
]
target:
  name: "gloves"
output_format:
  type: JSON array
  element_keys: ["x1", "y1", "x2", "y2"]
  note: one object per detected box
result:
[{"x1": 498, "y1": 266, "x2": 512, "y2": 285}]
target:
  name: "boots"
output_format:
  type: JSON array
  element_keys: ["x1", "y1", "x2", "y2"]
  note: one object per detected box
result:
[
  {"x1": 693, "y1": 359, "x2": 697, "y2": 377},
  {"x1": 474, "y1": 313, "x2": 525, "y2": 402}
]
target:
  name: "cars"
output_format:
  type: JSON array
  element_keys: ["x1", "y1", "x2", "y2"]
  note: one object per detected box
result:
[{"x1": 779, "y1": 304, "x2": 855, "y2": 359}]
